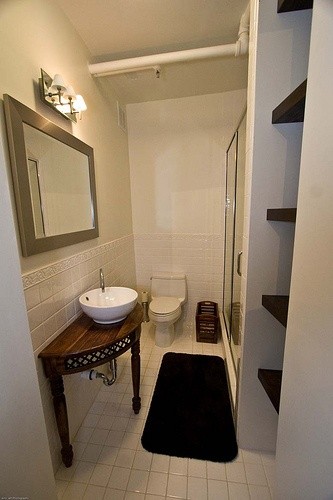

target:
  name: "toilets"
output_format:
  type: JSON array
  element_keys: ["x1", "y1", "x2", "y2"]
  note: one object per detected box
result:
[{"x1": 148, "y1": 274, "x2": 188, "y2": 347}]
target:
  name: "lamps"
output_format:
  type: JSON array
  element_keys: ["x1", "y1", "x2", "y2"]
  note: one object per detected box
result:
[{"x1": 38, "y1": 67, "x2": 88, "y2": 124}]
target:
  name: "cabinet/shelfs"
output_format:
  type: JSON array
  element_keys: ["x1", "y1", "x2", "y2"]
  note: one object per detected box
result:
[{"x1": 236, "y1": 0, "x2": 333, "y2": 500}]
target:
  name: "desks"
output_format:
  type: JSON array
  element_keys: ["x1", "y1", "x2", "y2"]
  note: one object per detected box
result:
[{"x1": 38, "y1": 300, "x2": 144, "y2": 469}]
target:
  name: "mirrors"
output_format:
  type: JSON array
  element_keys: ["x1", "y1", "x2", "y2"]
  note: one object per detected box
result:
[{"x1": 3, "y1": 92, "x2": 100, "y2": 257}]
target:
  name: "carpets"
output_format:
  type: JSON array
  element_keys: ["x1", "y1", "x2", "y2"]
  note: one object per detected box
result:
[{"x1": 140, "y1": 351, "x2": 238, "y2": 464}]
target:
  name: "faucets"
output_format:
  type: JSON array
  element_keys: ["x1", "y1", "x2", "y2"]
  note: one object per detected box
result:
[{"x1": 100, "y1": 268, "x2": 105, "y2": 292}]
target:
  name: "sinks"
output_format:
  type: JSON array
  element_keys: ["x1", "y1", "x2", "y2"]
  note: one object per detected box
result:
[{"x1": 79, "y1": 286, "x2": 138, "y2": 324}]
